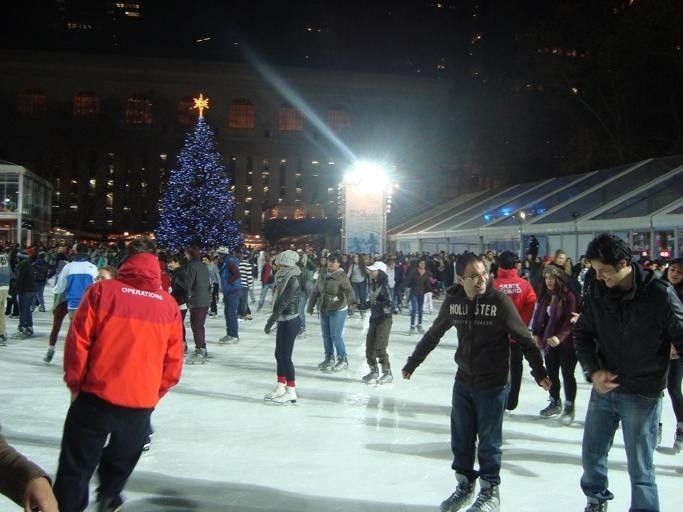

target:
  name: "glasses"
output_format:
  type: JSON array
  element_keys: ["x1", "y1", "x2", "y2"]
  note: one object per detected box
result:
[{"x1": 462, "y1": 270, "x2": 487, "y2": 280}]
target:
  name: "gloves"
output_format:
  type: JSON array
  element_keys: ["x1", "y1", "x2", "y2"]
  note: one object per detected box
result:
[{"x1": 264, "y1": 323, "x2": 270, "y2": 333}]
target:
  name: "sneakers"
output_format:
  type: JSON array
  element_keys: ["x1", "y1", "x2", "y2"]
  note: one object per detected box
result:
[
  {"x1": 0, "y1": 304, "x2": 45, "y2": 346},
  {"x1": 540, "y1": 395, "x2": 561, "y2": 416},
  {"x1": 42, "y1": 348, "x2": 54, "y2": 363},
  {"x1": 271, "y1": 385, "x2": 296, "y2": 403},
  {"x1": 294, "y1": 328, "x2": 305, "y2": 339},
  {"x1": 409, "y1": 326, "x2": 415, "y2": 336},
  {"x1": 561, "y1": 400, "x2": 574, "y2": 422},
  {"x1": 186, "y1": 311, "x2": 253, "y2": 365},
  {"x1": 584, "y1": 500, "x2": 607, "y2": 511},
  {"x1": 439, "y1": 469, "x2": 478, "y2": 512},
  {"x1": 317, "y1": 354, "x2": 393, "y2": 384},
  {"x1": 673, "y1": 420, "x2": 683, "y2": 450},
  {"x1": 266, "y1": 381, "x2": 286, "y2": 398},
  {"x1": 416, "y1": 324, "x2": 426, "y2": 335},
  {"x1": 464, "y1": 477, "x2": 500, "y2": 512}
]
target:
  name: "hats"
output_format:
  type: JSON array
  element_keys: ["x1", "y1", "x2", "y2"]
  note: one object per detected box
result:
[
  {"x1": 274, "y1": 249, "x2": 299, "y2": 267},
  {"x1": 542, "y1": 261, "x2": 570, "y2": 284},
  {"x1": 215, "y1": 247, "x2": 228, "y2": 255},
  {"x1": 365, "y1": 260, "x2": 387, "y2": 273}
]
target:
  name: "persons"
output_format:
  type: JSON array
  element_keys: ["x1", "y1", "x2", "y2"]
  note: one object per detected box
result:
[
  {"x1": 481, "y1": 250, "x2": 597, "y2": 426},
  {"x1": 158, "y1": 244, "x2": 459, "y2": 407},
  {"x1": 0, "y1": 238, "x2": 120, "y2": 366},
  {"x1": 49, "y1": 248, "x2": 184, "y2": 512},
  {"x1": 398, "y1": 251, "x2": 550, "y2": 511},
  {"x1": 638, "y1": 255, "x2": 683, "y2": 454},
  {"x1": 570, "y1": 233, "x2": 682, "y2": 512},
  {"x1": 0, "y1": 429, "x2": 64, "y2": 512}
]
